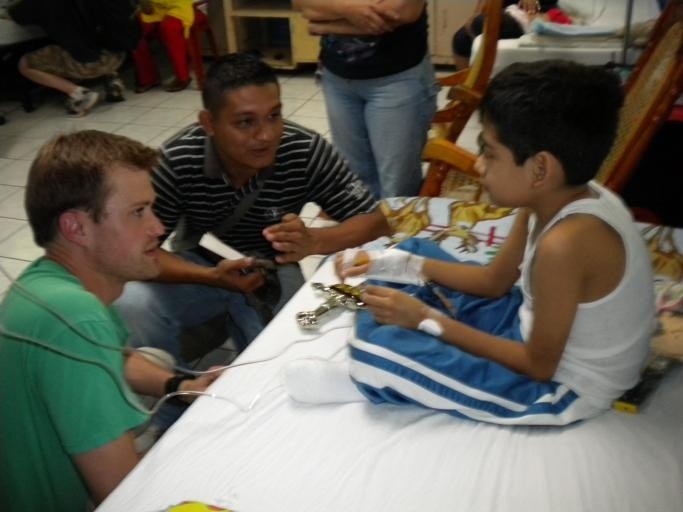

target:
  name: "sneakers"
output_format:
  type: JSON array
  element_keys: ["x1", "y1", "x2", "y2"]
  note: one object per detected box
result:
[
  {"x1": 165, "y1": 77, "x2": 191, "y2": 92},
  {"x1": 135, "y1": 79, "x2": 161, "y2": 93},
  {"x1": 105, "y1": 78, "x2": 125, "y2": 102},
  {"x1": 65, "y1": 89, "x2": 100, "y2": 118}
]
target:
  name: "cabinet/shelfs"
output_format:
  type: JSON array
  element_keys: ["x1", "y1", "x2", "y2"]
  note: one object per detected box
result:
[{"x1": 223, "y1": 4, "x2": 329, "y2": 70}]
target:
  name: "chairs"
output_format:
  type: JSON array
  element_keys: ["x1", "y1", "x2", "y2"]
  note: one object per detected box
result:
[{"x1": 416, "y1": 1, "x2": 681, "y2": 202}]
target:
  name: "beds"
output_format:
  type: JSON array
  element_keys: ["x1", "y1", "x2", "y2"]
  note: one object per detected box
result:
[{"x1": 86, "y1": 192, "x2": 682, "y2": 512}]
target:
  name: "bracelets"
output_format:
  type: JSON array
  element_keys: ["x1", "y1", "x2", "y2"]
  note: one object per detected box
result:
[{"x1": 163, "y1": 374, "x2": 194, "y2": 407}]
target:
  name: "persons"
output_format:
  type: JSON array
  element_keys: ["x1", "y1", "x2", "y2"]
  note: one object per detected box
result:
[
  {"x1": 124, "y1": 1, "x2": 209, "y2": 94},
  {"x1": 105, "y1": 51, "x2": 395, "y2": 437},
  {"x1": 333, "y1": 60, "x2": 658, "y2": 430},
  {"x1": 0, "y1": 128, "x2": 228, "y2": 511},
  {"x1": 295, "y1": 1, "x2": 439, "y2": 202},
  {"x1": 445, "y1": 1, "x2": 560, "y2": 113},
  {"x1": 4, "y1": 1, "x2": 141, "y2": 119}
]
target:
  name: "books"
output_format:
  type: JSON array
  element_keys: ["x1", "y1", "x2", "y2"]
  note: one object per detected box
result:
[
  {"x1": 605, "y1": 354, "x2": 679, "y2": 415},
  {"x1": 197, "y1": 232, "x2": 278, "y2": 272}
]
